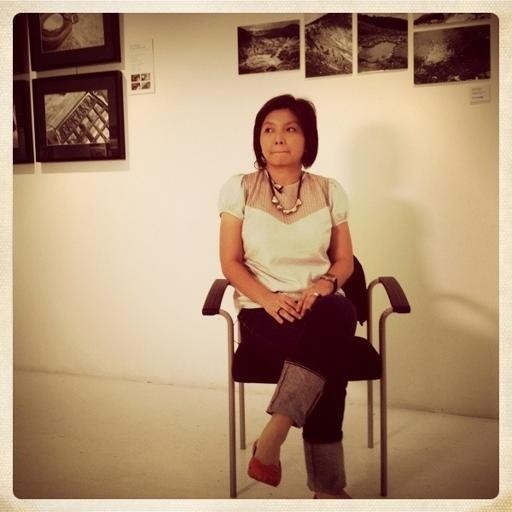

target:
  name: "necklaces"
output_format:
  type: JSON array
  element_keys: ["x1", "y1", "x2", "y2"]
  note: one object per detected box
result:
[{"x1": 266, "y1": 169, "x2": 303, "y2": 216}]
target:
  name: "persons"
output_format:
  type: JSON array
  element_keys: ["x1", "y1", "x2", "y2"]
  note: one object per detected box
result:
[{"x1": 219, "y1": 92, "x2": 358, "y2": 499}]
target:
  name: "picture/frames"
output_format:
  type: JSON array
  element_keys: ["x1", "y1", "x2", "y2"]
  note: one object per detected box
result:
[{"x1": 11, "y1": 12, "x2": 126, "y2": 165}]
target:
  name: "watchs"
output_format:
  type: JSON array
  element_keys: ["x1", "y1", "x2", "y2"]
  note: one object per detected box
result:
[{"x1": 319, "y1": 273, "x2": 338, "y2": 294}]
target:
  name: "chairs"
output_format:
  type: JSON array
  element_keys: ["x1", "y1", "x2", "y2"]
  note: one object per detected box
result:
[{"x1": 201, "y1": 252, "x2": 412, "y2": 499}]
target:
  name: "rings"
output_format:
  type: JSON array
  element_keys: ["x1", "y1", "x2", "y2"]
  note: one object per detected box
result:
[
  {"x1": 313, "y1": 292, "x2": 319, "y2": 297},
  {"x1": 276, "y1": 307, "x2": 283, "y2": 314}
]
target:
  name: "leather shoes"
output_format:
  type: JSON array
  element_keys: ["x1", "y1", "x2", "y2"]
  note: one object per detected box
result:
[{"x1": 247, "y1": 435, "x2": 283, "y2": 488}]
